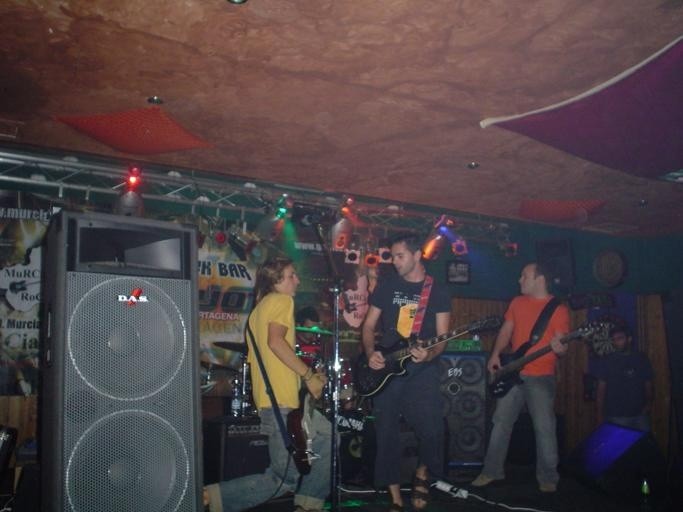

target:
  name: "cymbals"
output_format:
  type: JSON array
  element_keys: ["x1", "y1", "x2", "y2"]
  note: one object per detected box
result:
[
  {"x1": 200, "y1": 360, "x2": 240, "y2": 374},
  {"x1": 213, "y1": 342, "x2": 248, "y2": 354}
]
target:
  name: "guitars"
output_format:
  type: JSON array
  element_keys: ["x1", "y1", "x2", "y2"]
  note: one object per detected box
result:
[
  {"x1": 287, "y1": 353, "x2": 326, "y2": 474},
  {"x1": 486, "y1": 322, "x2": 604, "y2": 399},
  {"x1": 350, "y1": 314, "x2": 504, "y2": 399}
]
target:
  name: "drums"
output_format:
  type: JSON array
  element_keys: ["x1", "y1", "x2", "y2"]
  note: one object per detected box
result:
[
  {"x1": 331, "y1": 358, "x2": 365, "y2": 410},
  {"x1": 299, "y1": 342, "x2": 329, "y2": 385}
]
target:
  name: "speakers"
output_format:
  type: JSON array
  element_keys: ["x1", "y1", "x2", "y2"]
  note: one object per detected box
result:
[
  {"x1": 431, "y1": 350, "x2": 488, "y2": 468},
  {"x1": 212, "y1": 416, "x2": 271, "y2": 478},
  {"x1": 37, "y1": 210, "x2": 205, "y2": 508},
  {"x1": 574, "y1": 420, "x2": 665, "y2": 492}
]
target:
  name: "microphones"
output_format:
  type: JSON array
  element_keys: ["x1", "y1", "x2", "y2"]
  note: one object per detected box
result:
[{"x1": 327, "y1": 274, "x2": 347, "y2": 296}]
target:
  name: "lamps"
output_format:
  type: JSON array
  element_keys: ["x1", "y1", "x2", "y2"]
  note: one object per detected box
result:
[{"x1": 227, "y1": 229, "x2": 260, "y2": 253}]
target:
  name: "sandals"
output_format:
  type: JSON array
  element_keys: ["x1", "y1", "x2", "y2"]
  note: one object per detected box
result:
[
  {"x1": 387, "y1": 501, "x2": 402, "y2": 511},
  {"x1": 410, "y1": 475, "x2": 431, "y2": 511}
]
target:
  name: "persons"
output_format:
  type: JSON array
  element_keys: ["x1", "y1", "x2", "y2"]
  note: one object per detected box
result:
[
  {"x1": 294, "y1": 304, "x2": 350, "y2": 389},
  {"x1": 362, "y1": 234, "x2": 455, "y2": 511},
  {"x1": 200, "y1": 257, "x2": 339, "y2": 512},
  {"x1": 593, "y1": 325, "x2": 654, "y2": 435},
  {"x1": 469, "y1": 259, "x2": 570, "y2": 491}
]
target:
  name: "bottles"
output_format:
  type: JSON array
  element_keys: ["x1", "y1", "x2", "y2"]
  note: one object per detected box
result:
[
  {"x1": 240, "y1": 359, "x2": 252, "y2": 418},
  {"x1": 294, "y1": 344, "x2": 302, "y2": 355},
  {"x1": 635, "y1": 478, "x2": 652, "y2": 511},
  {"x1": 472, "y1": 334, "x2": 480, "y2": 352},
  {"x1": 230, "y1": 375, "x2": 242, "y2": 418}
]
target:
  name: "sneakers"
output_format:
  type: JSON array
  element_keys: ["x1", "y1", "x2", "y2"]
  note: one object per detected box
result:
[
  {"x1": 206, "y1": 483, "x2": 225, "y2": 512},
  {"x1": 471, "y1": 473, "x2": 495, "y2": 487},
  {"x1": 539, "y1": 481, "x2": 558, "y2": 493}
]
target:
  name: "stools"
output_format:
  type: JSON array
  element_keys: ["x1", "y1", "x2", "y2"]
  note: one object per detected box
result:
[{"x1": 13, "y1": 437, "x2": 38, "y2": 494}]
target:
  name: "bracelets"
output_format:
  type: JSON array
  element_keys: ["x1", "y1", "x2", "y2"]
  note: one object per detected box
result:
[{"x1": 299, "y1": 365, "x2": 315, "y2": 382}]
